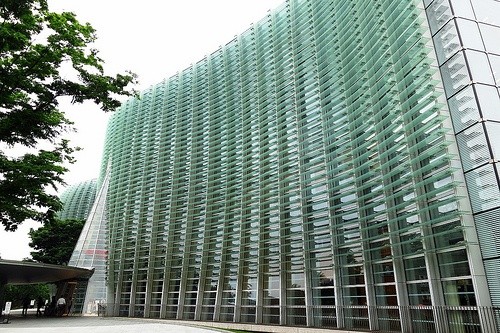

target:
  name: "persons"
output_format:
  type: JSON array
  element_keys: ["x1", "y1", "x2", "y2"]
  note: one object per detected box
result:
[
  {"x1": 1, "y1": 297, "x2": 12, "y2": 323},
  {"x1": 66, "y1": 296, "x2": 75, "y2": 317},
  {"x1": 35, "y1": 295, "x2": 44, "y2": 316},
  {"x1": 50, "y1": 295, "x2": 58, "y2": 318},
  {"x1": 56, "y1": 295, "x2": 66, "y2": 318},
  {"x1": 21, "y1": 294, "x2": 31, "y2": 316}
]
